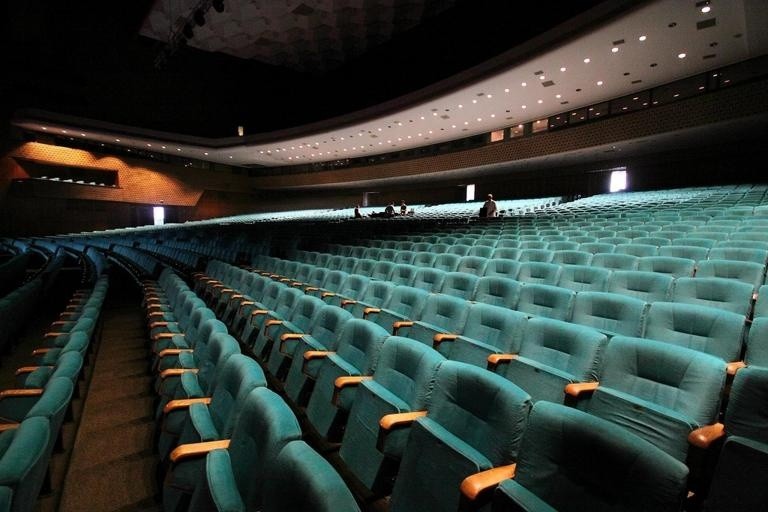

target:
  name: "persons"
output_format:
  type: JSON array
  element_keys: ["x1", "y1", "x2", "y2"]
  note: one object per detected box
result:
[
  {"x1": 479, "y1": 193, "x2": 498, "y2": 217},
  {"x1": 399, "y1": 198, "x2": 406, "y2": 215},
  {"x1": 353, "y1": 203, "x2": 363, "y2": 217},
  {"x1": 384, "y1": 200, "x2": 395, "y2": 217}
]
